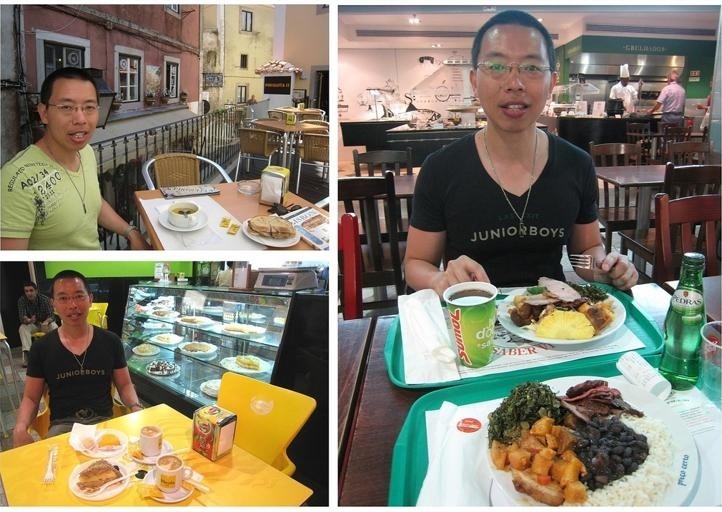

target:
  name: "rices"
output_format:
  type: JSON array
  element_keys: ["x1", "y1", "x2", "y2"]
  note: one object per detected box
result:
[{"x1": 562, "y1": 414, "x2": 672, "y2": 506}]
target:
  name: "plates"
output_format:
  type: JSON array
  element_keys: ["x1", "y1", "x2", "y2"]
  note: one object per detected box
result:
[
  {"x1": 67, "y1": 427, "x2": 196, "y2": 502},
  {"x1": 158, "y1": 209, "x2": 211, "y2": 233},
  {"x1": 497, "y1": 285, "x2": 627, "y2": 346},
  {"x1": 242, "y1": 217, "x2": 302, "y2": 247},
  {"x1": 486, "y1": 375, "x2": 700, "y2": 507},
  {"x1": 132, "y1": 310, "x2": 271, "y2": 398}
]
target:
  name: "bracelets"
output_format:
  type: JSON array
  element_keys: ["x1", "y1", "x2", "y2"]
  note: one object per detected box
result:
[
  {"x1": 127, "y1": 402, "x2": 144, "y2": 410},
  {"x1": 123, "y1": 225, "x2": 136, "y2": 239}
]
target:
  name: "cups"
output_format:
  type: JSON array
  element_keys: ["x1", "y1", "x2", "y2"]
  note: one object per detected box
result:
[
  {"x1": 443, "y1": 281, "x2": 499, "y2": 368},
  {"x1": 177, "y1": 272, "x2": 184, "y2": 281},
  {"x1": 700, "y1": 320, "x2": 722, "y2": 393},
  {"x1": 168, "y1": 200, "x2": 201, "y2": 228}
]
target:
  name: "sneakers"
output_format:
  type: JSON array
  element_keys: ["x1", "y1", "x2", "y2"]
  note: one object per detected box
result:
[{"x1": 22, "y1": 361, "x2": 28, "y2": 368}]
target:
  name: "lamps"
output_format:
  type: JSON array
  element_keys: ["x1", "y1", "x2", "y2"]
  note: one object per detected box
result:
[{"x1": 365, "y1": 89, "x2": 382, "y2": 121}]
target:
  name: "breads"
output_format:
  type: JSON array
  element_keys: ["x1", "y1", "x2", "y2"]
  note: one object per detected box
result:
[
  {"x1": 247, "y1": 214, "x2": 296, "y2": 240},
  {"x1": 224, "y1": 324, "x2": 249, "y2": 332},
  {"x1": 98, "y1": 433, "x2": 121, "y2": 448},
  {"x1": 184, "y1": 342, "x2": 210, "y2": 352},
  {"x1": 236, "y1": 355, "x2": 261, "y2": 370}
]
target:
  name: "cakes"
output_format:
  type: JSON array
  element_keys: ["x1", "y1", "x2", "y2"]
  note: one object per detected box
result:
[
  {"x1": 146, "y1": 360, "x2": 176, "y2": 376},
  {"x1": 203, "y1": 380, "x2": 220, "y2": 397},
  {"x1": 76, "y1": 461, "x2": 125, "y2": 494}
]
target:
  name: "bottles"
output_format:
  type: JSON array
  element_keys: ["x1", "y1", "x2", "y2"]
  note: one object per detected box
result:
[
  {"x1": 162, "y1": 263, "x2": 169, "y2": 287},
  {"x1": 657, "y1": 252, "x2": 708, "y2": 391}
]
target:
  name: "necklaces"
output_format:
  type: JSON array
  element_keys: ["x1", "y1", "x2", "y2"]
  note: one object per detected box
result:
[
  {"x1": 42, "y1": 139, "x2": 86, "y2": 214},
  {"x1": 59, "y1": 326, "x2": 90, "y2": 371},
  {"x1": 482, "y1": 126, "x2": 537, "y2": 237}
]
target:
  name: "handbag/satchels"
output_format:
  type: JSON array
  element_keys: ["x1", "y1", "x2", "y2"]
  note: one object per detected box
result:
[{"x1": 700, "y1": 112, "x2": 711, "y2": 132}]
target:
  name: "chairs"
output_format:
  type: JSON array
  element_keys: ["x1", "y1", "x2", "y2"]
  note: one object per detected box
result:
[
  {"x1": 218, "y1": 370, "x2": 317, "y2": 476},
  {"x1": 142, "y1": 153, "x2": 232, "y2": 190},
  {"x1": 22, "y1": 296, "x2": 110, "y2": 367},
  {"x1": 26, "y1": 380, "x2": 127, "y2": 441},
  {"x1": 232, "y1": 106, "x2": 329, "y2": 196}
]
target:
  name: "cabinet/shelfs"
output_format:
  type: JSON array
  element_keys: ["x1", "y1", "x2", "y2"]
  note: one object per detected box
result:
[{"x1": 122, "y1": 283, "x2": 291, "y2": 422}]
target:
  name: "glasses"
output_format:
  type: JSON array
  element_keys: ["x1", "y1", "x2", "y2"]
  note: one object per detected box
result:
[
  {"x1": 46, "y1": 102, "x2": 102, "y2": 115},
  {"x1": 54, "y1": 293, "x2": 89, "y2": 304},
  {"x1": 475, "y1": 59, "x2": 552, "y2": 79},
  {"x1": 266, "y1": 203, "x2": 302, "y2": 216}
]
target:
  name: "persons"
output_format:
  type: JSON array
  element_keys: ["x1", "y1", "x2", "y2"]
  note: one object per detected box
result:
[
  {"x1": 246, "y1": 94, "x2": 257, "y2": 105},
  {"x1": 609, "y1": 63, "x2": 644, "y2": 119},
  {"x1": 215, "y1": 262, "x2": 234, "y2": 288},
  {"x1": 17, "y1": 281, "x2": 59, "y2": 367},
  {"x1": 695, "y1": 95, "x2": 712, "y2": 129},
  {"x1": 647, "y1": 70, "x2": 686, "y2": 166},
  {"x1": 0, "y1": 66, "x2": 153, "y2": 250},
  {"x1": 13, "y1": 269, "x2": 145, "y2": 448},
  {"x1": 403, "y1": 11, "x2": 639, "y2": 301}
]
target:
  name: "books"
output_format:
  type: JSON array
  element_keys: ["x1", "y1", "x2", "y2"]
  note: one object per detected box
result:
[{"x1": 280, "y1": 206, "x2": 330, "y2": 250}]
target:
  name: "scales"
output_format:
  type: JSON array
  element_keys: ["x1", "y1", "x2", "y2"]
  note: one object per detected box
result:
[{"x1": 253, "y1": 267, "x2": 319, "y2": 292}]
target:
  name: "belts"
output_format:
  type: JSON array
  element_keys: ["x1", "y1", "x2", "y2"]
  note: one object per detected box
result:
[
  {"x1": 663, "y1": 111, "x2": 684, "y2": 116},
  {"x1": 51, "y1": 407, "x2": 113, "y2": 419}
]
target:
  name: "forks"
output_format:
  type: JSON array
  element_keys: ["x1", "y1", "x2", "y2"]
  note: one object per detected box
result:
[
  {"x1": 42, "y1": 450, "x2": 56, "y2": 486},
  {"x1": 570, "y1": 254, "x2": 615, "y2": 272}
]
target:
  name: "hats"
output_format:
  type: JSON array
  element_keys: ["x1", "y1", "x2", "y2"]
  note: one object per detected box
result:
[{"x1": 619, "y1": 64, "x2": 629, "y2": 78}]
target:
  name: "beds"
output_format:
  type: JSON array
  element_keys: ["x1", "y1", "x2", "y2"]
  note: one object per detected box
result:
[{"x1": 0, "y1": 403, "x2": 314, "y2": 506}]
